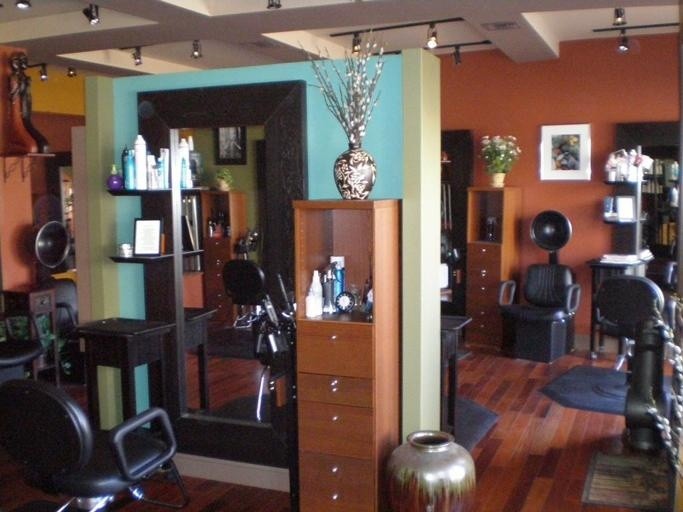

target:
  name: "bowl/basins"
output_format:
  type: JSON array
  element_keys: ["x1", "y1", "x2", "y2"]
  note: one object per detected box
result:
[{"x1": 23, "y1": 221, "x2": 71, "y2": 269}]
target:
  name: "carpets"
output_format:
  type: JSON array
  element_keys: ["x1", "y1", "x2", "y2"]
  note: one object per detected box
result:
[
  {"x1": 441, "y1": 349, "x2": 675, "y2": 511},
  {"x1": 185, "y1": 307, "x2": 294, "y2": 444},
  {"x1": 11, "y1": 500, "x2": 90, "y2": 512}
]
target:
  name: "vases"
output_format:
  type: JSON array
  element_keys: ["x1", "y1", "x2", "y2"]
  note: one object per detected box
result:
[
  {"x1": 385, "y1": 430, "x2": 476, "y2": 512},
  {"x1": 491, "y1": 171, "x2": 506, "y2": 187},
  {"x1": 333, "y1": 143, "x2": 377, "y2": 200}
]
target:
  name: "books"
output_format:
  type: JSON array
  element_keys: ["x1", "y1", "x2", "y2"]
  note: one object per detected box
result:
[{"x1": 642, "y1": 157, "x2": 680, "y2": 289}]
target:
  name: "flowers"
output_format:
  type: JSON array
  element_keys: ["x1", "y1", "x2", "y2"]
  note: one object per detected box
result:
[
  {"x1": 299, "y1": 26, "x2": 388, "y2": 144},
  {"x1": 478, "y1": 134, "x2": 522, "y2": 174}
]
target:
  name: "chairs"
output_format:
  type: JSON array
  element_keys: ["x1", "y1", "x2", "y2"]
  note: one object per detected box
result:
[
  {"x1": 0, "y1": 377, "x2": 190, "y2": 512},
  {"x1": 489, "y1": 208, "x2": 681, "y2": 400},
  {"x1": 223, "y1": 260, "x2": 265, "y2": 330}
]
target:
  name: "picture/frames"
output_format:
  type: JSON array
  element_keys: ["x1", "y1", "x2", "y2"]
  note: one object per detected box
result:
[
  {"x1": 44, "y1": 151, "x2": 75, "y2": 243},
  {"x1": 616, "y1": 196, "x2": 636, "y2": 223},
  {"x1": 541, "y1": 123, "x2": 591, "y2": 182},
  {"x1": 214, "y1": 126, "x2": 248, "y2": 165},
  {"x1": 134, "y1": 217, "x2": 165, "y2": 257}
]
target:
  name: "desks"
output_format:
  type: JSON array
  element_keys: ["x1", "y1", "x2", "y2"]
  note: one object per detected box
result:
[
  {"x1": 0, "y1": 285, "x2": 177, "y2": 465},
  {"x1": 441, "y1": 316, "x2": 472, "y2": 433}
]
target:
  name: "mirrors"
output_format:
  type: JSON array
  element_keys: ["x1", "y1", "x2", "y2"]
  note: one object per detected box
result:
[
  {"x1": 136, "y1": 79, "x2": 309, "y2": 469},
  {"x1": 614, "y1": 123, "x2": 680, "y2": 291},
  {"x1": 441, "y1": 128, "x2": 473, "y2": 315}
]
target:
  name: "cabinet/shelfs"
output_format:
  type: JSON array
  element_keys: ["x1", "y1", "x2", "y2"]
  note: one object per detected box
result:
[
  {"x1": 604, "y1": 181, "x2": 636, "y2": 226},
  {"x1": 107, "y1": 189, "x2": 173, "y2": 263},
  {"x1": 203, "y1": 191, "x2": 247, "y2": 324},
  {"x1": 290, "y1": 199, "x2": 403, "y2": 511},
  {"x1": 466, "y1": 186, "x2": 523, "y2": 349},
  {"x1": 181, "y1": 187, "x2": 210, "y2": 255}
]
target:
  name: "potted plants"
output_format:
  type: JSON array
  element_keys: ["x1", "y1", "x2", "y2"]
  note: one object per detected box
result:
[{"x1": 212, "y1": 167, "x2": 235, "y2": 192}]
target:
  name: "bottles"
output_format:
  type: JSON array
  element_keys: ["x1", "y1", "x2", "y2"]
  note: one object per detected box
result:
[
  {"x1": 671, "y1": 187, "x2": 677, "y2": 203},
  {"x1": 121, "y1": 144, "x2": 135, "y2": 191},
  {"x1": 148, "y1": 146, "x2": 168, "y2": 190},
  {"x1": 668, "y1": 159, "x2": 678, "y2": 180},
  {"x1": 179, "y1": 139, "x2": 189, "y2": 172},
  {"x1": 134, "y1": 135, "x2": 147, "y2": 190},
  {"x1": 107, "y1": 164, "x2": 124, "y2": 191},
  {"x1": 208, "y1": 210, "x2": 225, "y2": 238},
  {"x1": 180, "y1": 160, "x2": 188, "y2": 189},
  {"x1": 306, "y1": 255, "x2": 345, "y2": 319}
]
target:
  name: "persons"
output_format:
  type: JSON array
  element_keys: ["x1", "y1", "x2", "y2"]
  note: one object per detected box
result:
[{"x1": 219, "y1": 128, "x2": 240, "y2": 158}]
